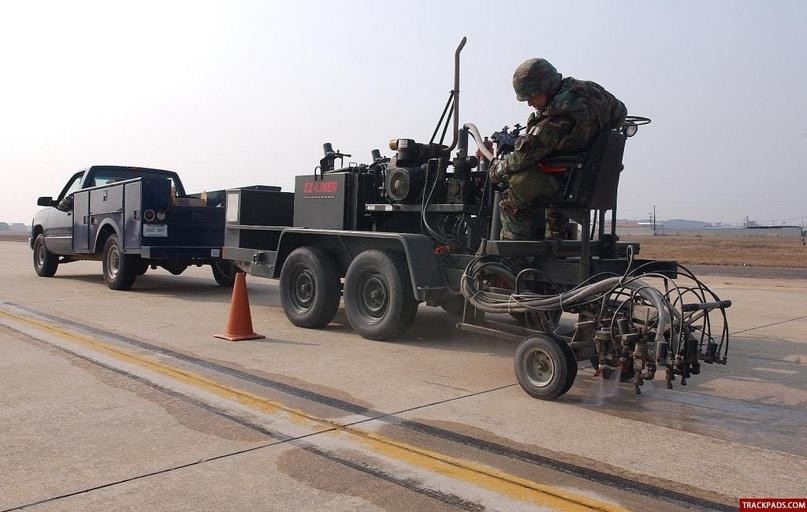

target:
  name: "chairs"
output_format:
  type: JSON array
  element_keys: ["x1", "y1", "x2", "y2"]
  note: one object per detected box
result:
[{"x1": 528, "y1": 130, "x2": 627, "y2": 237}]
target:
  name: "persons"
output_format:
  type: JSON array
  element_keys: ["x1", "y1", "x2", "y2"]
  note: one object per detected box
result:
[{"x1": 486, "y1": 56, "x2": 626, "y2": 241}]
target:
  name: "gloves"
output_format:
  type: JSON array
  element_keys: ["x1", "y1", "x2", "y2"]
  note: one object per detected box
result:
[{"x1": 487, "y1": 160, "x2": 510, "y2": 183}]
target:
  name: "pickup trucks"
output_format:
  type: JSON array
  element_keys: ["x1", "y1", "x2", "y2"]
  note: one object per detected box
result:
[{"x1": 28, "y1": 165, "x2": 283, "y2": 291}]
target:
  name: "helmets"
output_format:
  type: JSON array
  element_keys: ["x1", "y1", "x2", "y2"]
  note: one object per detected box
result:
[{"x1": 512, "y1": 58, "x2": 561, "y2": 102}]
